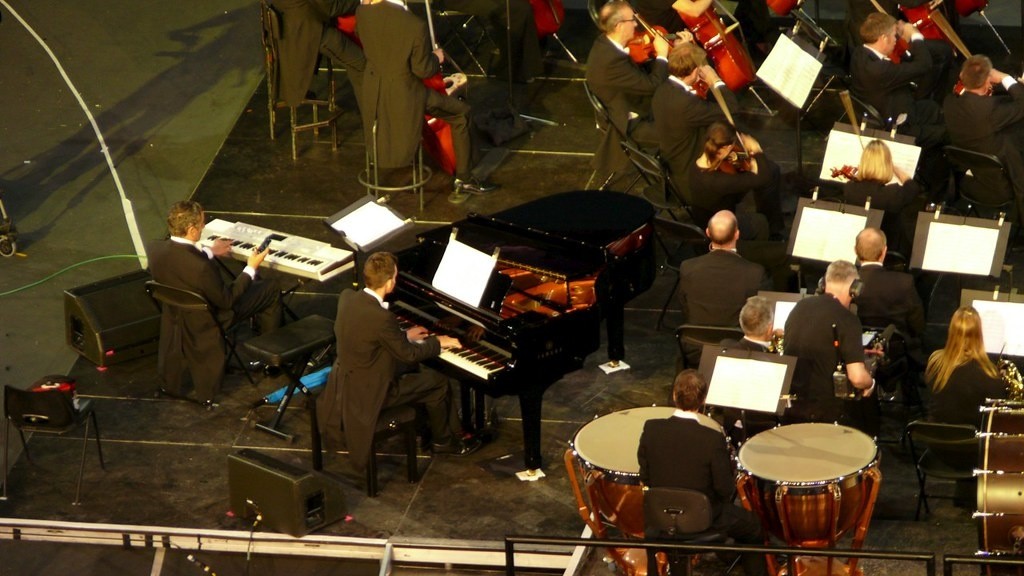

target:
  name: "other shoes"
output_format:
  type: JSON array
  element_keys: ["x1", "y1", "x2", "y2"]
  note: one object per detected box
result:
[
  {"x1": 264, "y1": 364, "x2": 281, "y2": 378},
  {"x1": 248, "y1": 359, "x2": 264, "y2": 373}
]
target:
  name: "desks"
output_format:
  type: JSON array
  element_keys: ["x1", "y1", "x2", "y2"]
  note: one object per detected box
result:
[{"x1": 225, "y1": 315, "x2": 341, "y2": 438}]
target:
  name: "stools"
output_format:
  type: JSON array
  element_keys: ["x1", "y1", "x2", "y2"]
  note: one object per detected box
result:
[
  {"x1": 356, "y1": 114, "x2": 434, "y2": 213},
  {"x1": 345, "y1": 408, "x2": 426, "y2": 498}
]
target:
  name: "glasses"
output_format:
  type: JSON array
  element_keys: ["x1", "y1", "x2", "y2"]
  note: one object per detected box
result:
[{"x1": 615, "y1": 15, "x2": 635, "y2": 27}]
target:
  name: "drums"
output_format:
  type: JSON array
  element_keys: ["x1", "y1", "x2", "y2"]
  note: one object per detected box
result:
[
  {"x1": 971, "y1": 398, "x2": 1024, "y2": 576},
  {"x1": 567, "y1": 406, "x2": 736, "y2": 575},
  {"x1": 734, "y1": 420, "x2": 882, "y2": 576}
]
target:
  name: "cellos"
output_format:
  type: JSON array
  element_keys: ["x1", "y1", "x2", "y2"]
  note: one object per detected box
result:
[
  {"x1": 955, "y1": 0, "x2": 1011, "y2": 55},
  {"x1": 420, "y1": 72, "x2": 457, "y2": 177},
  {"x1": 766, "y1": 0, "x2": 816, "y2": 24},
  {"x1": 529, "y1": 0, "x2": 578, "y2": 63},
  {"x1": 674, "y1": 0, "x2": 775, "y2": 116}
]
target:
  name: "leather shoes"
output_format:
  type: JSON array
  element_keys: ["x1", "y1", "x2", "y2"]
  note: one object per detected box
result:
[
  {"x1": 450, "y1": 174, "x2": 501, "y2": 195},
  {"x1": 432, "y1": 436, "x2": 480, "y2": 457}
]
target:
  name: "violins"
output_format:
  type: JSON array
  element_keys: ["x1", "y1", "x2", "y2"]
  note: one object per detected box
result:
[
  {"x1": 691, "y1": 73, "x2": 710, "y2": 98},
  {"x1": 716, "y1": 146, "x2": 754, "y2": 174},
  {"x1": 626, "y1": 22, "x2": 705, "y2": 64},
  {"x1": 831, "y1": 165, "x2": 864, "y2": 183},
  {"x1": 952, "y1": 80, "x2": 994, "y2": 97}
]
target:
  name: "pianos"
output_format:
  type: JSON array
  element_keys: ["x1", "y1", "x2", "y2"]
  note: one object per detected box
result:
[
  {"x1": 192, "y1": 218, "x2": 356, "y2": 371},
  {"x1": 353, "y1": 189, "x2": 713, "y2": 472}
]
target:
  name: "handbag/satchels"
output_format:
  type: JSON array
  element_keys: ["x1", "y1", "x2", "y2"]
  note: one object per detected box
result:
[{"x1": 473, "y1": 99, "x2": 529, "y2": 146}]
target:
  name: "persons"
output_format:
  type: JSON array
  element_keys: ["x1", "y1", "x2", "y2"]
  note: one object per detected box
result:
[
  {"x1": 148, "y1": 201, "x2": 283, "y2": 373},
  {"x1": 318, "y1": 251, "x2": 484, "y2": 469},
  {"x1": 271, "y1": 0, "x2": 1024, "y2": 507}
]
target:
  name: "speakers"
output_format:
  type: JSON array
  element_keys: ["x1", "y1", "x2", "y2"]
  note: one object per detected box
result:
[
  {"x1": 228, "y1": 448, "x2": 363, "y2": 537},
  {"x1": 63, "y1": 268, "x2": 163, "y2": 368}
]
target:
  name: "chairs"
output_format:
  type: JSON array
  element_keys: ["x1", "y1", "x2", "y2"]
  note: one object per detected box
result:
[
  {"x1": 579, "y1": 79, "x2": 626, "y2": 140},
  {"x1": 142, "y1": 278, "x2": 256, "y2": 387},
  {"x1": 653, "y1": 214, "x2": 712, "y2": 328},
  {"x1": 929, "y1": 142, "x2": 1024, "y2": 230},
  {"x1": 785, "y1": 8, "x2": 841, "y2": 66},
  {"x1": 839, "y1": 88, "x2": 891, "y2": 134},
  {"x1": 621, "y1": 142, "x2": 690, "y2": 228},
  {"x1": 1, "y1": 381, "x2": 104, "y2": 512},
  {"x1": 434, "y1": 9, "x2": 499, "y2": 82},
  {"x1": 551, "y1": 30, "x2": 586, "y2": 70},
  {"x1": 640, "y1": 487, "x2": 725, "y2": 576},
  {"x1": 250, "y1": 0, "x2": 344, "y2": 163},
  {"x1": 900, "y1": 416, "x2": 983, "y2": 527}
]
